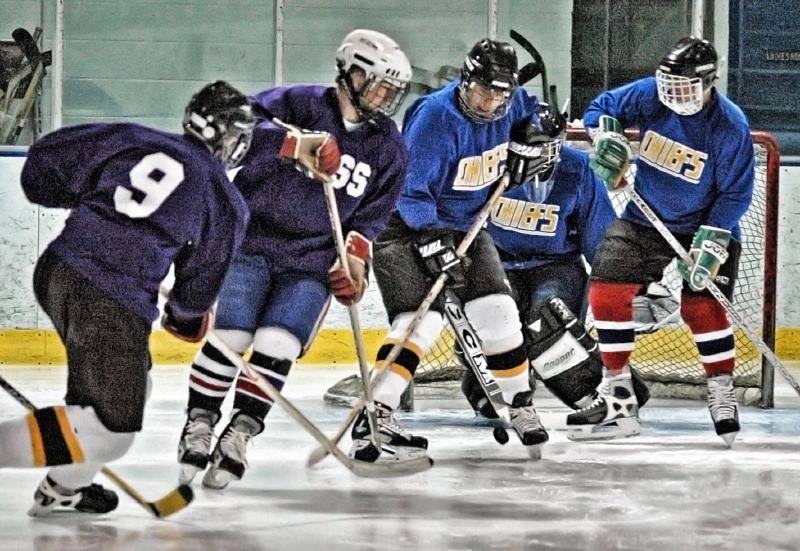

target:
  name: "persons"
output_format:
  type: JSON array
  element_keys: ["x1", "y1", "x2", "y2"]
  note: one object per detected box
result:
[
  {"x1": 349, "y1": 38, "x2": 650, "y2": 464},
  {"x1": 179, "y1": 29, "x2": 413, "y2": 489},
  {"x1": 0, "y1": 80, "x2": 256, "y2": 517},
  {"x1": 567, "y1": 36, "x2": 755, "y2": 446}
]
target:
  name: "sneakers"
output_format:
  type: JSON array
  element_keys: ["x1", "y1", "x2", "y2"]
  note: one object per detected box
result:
[
  {"x1": 348, "y1": 401, "x2": 428, "y2": 463},
  {"x1": 27, "y1": 474, "x2": 118, "y2": 517},
  {"x1": 178, "y1": 406, "x2": 222, "y2": 485},
  {"x1": 202, "y1": 410, "x2": 265, "y2": 489},
  {"x1": 706, "y1": 371, "x2": 741, "y2": 448},
  {"x1": 508, "y1": 405, "x2": 549, "y2": 459},
  {"x1": 566, "y1": 366, "x2": 641, "y2": 441}
]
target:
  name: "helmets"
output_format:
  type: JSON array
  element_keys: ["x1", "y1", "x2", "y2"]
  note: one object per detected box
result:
[
  {"x1": 335, "y1": 29, "x2": 411, "y2": 121},
  {"x1": 460, "y1": 38, "x2": 518, "y2": 123},
  {"x1": 658, "y1": 37, "x2": 720, "y2": 116},
  {"x1": 182, "y1": 80, "x2": 257, "y2": 159},
  {"x1": 531, "y1": 103, "x2": 568, "y2": 180}
]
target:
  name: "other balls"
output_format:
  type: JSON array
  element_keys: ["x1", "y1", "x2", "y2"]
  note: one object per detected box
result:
[{"x1": 493, "y1": 424, "x2": 508, "y2": 446}]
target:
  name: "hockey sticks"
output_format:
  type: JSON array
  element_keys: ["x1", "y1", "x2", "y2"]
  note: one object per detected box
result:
[
  {"x1": 0, "y1": 375, "x2": 193, "y2": 520},
  {"x1": 617, "y1": 178, "x2": 800, "y2": 398},
  {"x1": 307, "y1": 169, "x2": 515, "y2": 468},
  {"x1": 321, "y1": 178, "x2": 425, "y2": 463},
  {"x1": 159, "y1": 284, "x2": 435, "y2": 480}
]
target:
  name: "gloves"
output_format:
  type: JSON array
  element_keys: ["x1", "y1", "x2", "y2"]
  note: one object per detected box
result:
[
  {"x1": 273, "y1": 118, "x2": 341, "y2": 183},
  {"x1": 417, "y1": 225, "x2": 470, "y2": 289},
  {"x1": 326, "y1": 231, "x2": 373, "y2": 306},
  {"x1": 677, "y1": 225, "x2": 731, "y2": 291},
  {"x1": 587, "y1": 115, "x2": 633, "y2": 191},
  {"x1": 505, "y1": 122, "x2": 546, "y2": 190}
]
